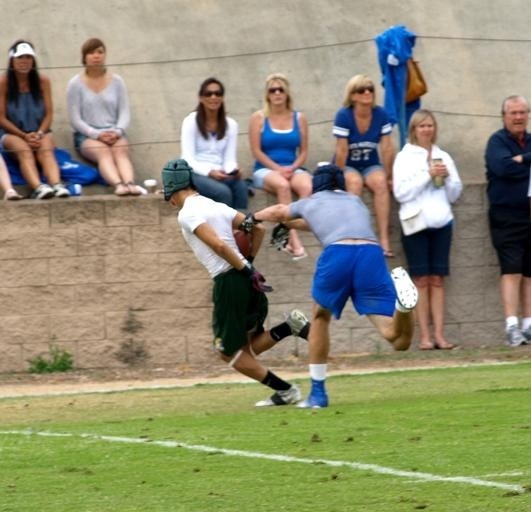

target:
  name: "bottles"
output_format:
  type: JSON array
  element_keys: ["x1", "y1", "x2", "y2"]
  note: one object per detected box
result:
[{"x1": 431, "y1": 158, "x2": 447, "y2": 188}]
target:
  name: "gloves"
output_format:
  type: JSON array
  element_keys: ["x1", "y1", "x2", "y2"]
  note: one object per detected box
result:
[
  {"x1": 269, "y1": 222, "x2": 289, "y2": 252},
  {"x1": 241, "y1": 263, "x2": 274, "y2": 294},
  {"x1": 236, "y1": 212, "x2": 264, "y2": 234}
]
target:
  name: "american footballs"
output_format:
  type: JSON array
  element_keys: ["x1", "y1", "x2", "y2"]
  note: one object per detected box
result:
[{"x1": 232, "y1": 229, "x2": 252, "y2": 259}]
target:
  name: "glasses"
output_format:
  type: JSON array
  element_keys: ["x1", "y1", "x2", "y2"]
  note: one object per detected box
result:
[
  {"x1": 351, "y1": 85, "x2": 375, "y2": 92},
  {"x1": 269, "y1": 86, "x2": 284, "y2": 94},
  {"x1": 201, "y1": 90, "x2": 224, "y2": 97}
]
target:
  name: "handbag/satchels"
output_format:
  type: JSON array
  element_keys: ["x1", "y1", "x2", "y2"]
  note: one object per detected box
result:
[
  {"x1": 404, "y1": 58, "x2": 428, "y2": 102},
  {"x1": 397, "y1": 203, "x2": 427, "y2": 238}
]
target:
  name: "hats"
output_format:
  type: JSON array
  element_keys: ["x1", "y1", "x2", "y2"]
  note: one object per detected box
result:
[{"x1": 8, "y1": 41, "x2": 36, "y2": 59}]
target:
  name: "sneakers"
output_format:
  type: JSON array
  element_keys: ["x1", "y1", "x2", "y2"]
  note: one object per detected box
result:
[
  {"x1": 503, "y1": 324, "x2": 528, "y2": 348},
  {"x1": 390, "y1": 265, "x2": 419, "y2": 311},
  {"x1": 297, "y1": 376, "x2": 329, "y2": 408},
  {"x1": 253, "y1": 384, "x2": 302, "y2": 407},
  {"x1": 286, "y1": 308, "x2": 312, "y2": 342},
  {"x1": 521, "y1": 324, "x2": 531, "y2": 340},
  {"x1": 51, "y1": 183, "x2": 70, "y2": 197},
  {"x1": 33, "y1": 182, "x2": 54, "y2": 199},
  {"x1": 6, "y1": 188, "x2": 21, "y2": 199}
]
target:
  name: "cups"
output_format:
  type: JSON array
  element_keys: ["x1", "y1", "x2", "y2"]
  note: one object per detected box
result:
[{"x1": 144, "y1": 180, "x2": 157, "y2": 193}]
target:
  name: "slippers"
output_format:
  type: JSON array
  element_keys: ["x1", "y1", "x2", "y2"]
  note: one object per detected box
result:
[
  {"x1": 112, "y1": 182, "x2": 129, "y2": 195},
  {"x1": 282, "y1": 243, "x2": 308, "y2": 262},
  {"x1": 125, "y1": 182, "x2": 142, "y2": 196}
]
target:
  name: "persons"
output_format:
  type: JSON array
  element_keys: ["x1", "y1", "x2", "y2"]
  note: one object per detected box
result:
[
  {"x1": 66, "y1": 37, "x2": 142, "y2": 195},
  {"x1": 163, "y1": 158, "x2": 309, "y2": 407},
  {"x1": 179, "y1": 77, "x2": 249, "y2": 214},
  {"x1": 247, "y1": 71, "x2": 314, "y2": 260},
  {"x1": 329, "y1": 73, "x2": 399, "y2": 258},
  {"x1": 0, "y1": 153, "x2": 21, "y2": 200},
  {"x1": 484, "y1": 93, "x2": 531, "y2": 347},
  {"x1": 0, "y1": 38, "x2": 72, "y2": 198},
  {"x1": 392, "y1": 109, "x2": 459, "y2": 349},
  {"x1": 240, "y1": 160, "x2": 419, "y2": 408}
]
